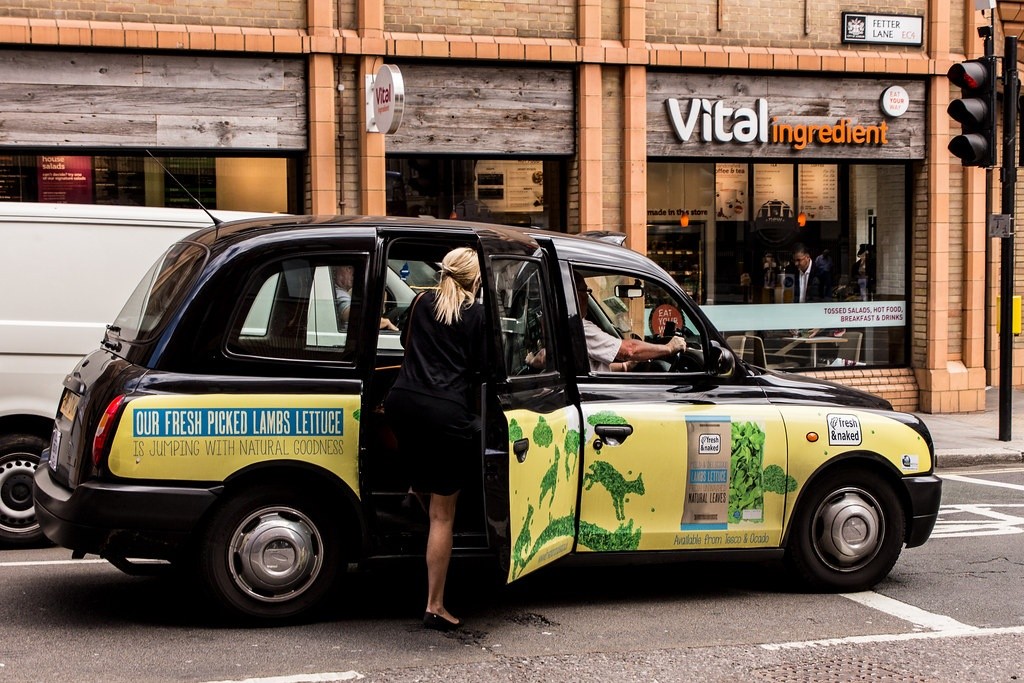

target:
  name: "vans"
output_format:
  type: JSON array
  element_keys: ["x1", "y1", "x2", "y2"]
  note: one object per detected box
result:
[{"x1": 0, "y1": 202, "x2": 416, "y2": 549}]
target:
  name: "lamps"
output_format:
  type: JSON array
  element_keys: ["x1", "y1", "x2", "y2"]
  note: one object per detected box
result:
[
  {"x1": 798, "y1": 161, "x2": 806, "y2": 226},
  {"x1": 681, "y1": 162, "x2": 689, "y2": 227}
]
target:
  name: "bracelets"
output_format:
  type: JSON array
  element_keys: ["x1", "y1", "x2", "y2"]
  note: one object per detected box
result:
[{"x1": 622, "y1": 362, "x2": 627, "y2": 372}]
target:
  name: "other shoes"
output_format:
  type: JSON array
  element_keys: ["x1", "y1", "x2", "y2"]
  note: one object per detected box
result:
[
  {"x1": 423, "y1": 612, "x2": 464, "y2": 630},
  {"x1": 400, "y1": 494, "x2": 429, "y2": 522}
]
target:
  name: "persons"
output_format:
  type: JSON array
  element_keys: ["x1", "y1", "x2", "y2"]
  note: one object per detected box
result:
[
  {"x1": 815, "y1": 249, "x2": 834, "y2": 298},
  {"x1": 572, "y1": 270, "x2": 686, "y2": 372},
  {"x1": 333, "y1": 266, "x2": 399, "y2": 331},
  {"x1": 379, "y1": 247, "x2": 547, "y2": 630},
  {"x1": 789, "y1": 243, "x2": 847, "y2": 364}
]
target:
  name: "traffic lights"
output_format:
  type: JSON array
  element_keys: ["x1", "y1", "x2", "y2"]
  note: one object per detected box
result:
[{"x1": 946, "y1": 57, "x2": 998, "y2": 168}]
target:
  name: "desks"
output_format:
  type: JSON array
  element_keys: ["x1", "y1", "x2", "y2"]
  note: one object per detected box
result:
[{"x1": 783, "y1": 337, "x2": 848, "y2": 367}]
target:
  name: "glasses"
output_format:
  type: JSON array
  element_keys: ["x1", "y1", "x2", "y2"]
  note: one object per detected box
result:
[{"x1": 576, "y1": 289, "x2": 592, "y2": 296}]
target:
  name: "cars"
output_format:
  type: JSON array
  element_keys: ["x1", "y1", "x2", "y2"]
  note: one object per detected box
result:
[{"x1": 31, "y1": 214, "x2": 943, "y2": 627}]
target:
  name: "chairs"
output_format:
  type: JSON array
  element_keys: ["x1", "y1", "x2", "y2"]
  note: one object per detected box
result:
[
  {"x1": 838, "y1": 332, "x2": 863, "y2": 362},
  {"x1": 726, "y1": 336, "x2": 746, "y2": 362},
  {"x1": 745, "y1": 335, "x2": 799, "y2": 370}
]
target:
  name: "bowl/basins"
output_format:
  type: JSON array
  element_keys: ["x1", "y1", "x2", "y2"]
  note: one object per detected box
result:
[{"x1": 800, "y1": 329, "x2": 814, "y2": 338}]
